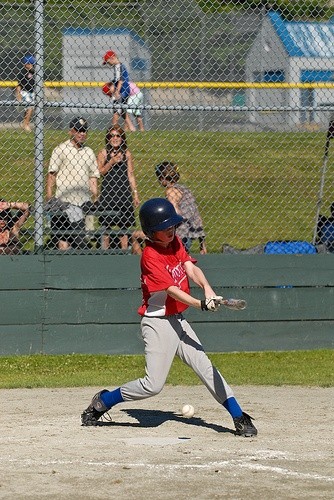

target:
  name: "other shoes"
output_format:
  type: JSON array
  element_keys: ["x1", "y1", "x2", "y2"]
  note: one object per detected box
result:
[{"x1": 19, "y1": 124, "x2": 32, "y2": 132}]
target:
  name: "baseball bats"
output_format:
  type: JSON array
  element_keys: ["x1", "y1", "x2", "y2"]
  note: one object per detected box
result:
[{"x1": 213, "y1": 297, "x2": 247, "y2": 311}]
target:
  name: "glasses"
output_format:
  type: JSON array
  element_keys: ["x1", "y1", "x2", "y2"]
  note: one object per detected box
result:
[{"x1": 109, "y1": 134, "x2": 123, "y2": 138}]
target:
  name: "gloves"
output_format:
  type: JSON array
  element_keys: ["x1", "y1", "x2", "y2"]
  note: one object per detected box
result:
[{"x1": 201, "y1": 295, "x2": 223, "y2": 312}]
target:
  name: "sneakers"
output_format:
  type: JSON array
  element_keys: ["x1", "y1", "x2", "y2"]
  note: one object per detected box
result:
[
  {"x1": 81, "y1": 389, "x2": 112, "y2": 426},
  {"x1": 233, "y1": 412, "x2": 258, "y2": 437}
]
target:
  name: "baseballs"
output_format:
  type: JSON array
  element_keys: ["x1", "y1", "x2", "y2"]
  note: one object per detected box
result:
[{"x1": 182, "y1": 404, "x2": 195, "y2": 419}]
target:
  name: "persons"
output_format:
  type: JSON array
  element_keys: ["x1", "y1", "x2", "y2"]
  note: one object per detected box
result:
[
  {"x1": 0, "y1": 200, "x2": 30, "y2": 255},
  {"x1": 102, "y1": 80, "x2": 145, "y2": 131},
  {"x1": 47, "y1": 117, "x2": 100, "y2": 250},
  {"x1": 80, "y1": 198, "x2": 258, "y2": 437},
  {"x1": 16, "y1": 56, "x2": 35, "y2": 133},
  {"x1": 96, "y1": 126, "x2": 140, "y2": 251},
  {"x1": 102, "y1": 51, "x2": 130, "y2": 131},
  {"x1": 155, "y1": 162, "x2": 208, "y2": 255}
]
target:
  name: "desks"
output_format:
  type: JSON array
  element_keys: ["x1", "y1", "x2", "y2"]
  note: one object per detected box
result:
[{"x1": 29, "y1": 210, "x2": 121, "y2": 250}]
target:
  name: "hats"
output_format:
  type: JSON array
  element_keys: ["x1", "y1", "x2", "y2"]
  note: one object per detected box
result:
[
  {"x1": 102, "y1": 50, "x2": 115, "y2": 65},
  {"x1": 102, "y1": 82, "x2": 112, "y2": 98},
  {"x1": 23, "y1": 56, "x2": 36, "y2": 64},
  {"x1": 70, "y1": 117, "x2": 88, "y2": 129}
]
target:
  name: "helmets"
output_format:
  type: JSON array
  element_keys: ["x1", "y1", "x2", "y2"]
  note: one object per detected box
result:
[{"x1": 139, "y1": 198, "x2": 184, "y2": 239}]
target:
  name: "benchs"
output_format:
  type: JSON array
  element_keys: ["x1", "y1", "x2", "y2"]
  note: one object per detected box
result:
[
  {"x1": 22, "y1": 248, "x2": 132, "y2": 255},
  {"x1": 19, "y1": 229, "x2": 135, "y2": 237}
]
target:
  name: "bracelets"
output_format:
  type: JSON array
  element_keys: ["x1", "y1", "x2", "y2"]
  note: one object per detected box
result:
[{"x1": 131, "y1": 190, "x2": 137, "y2": 195}]
